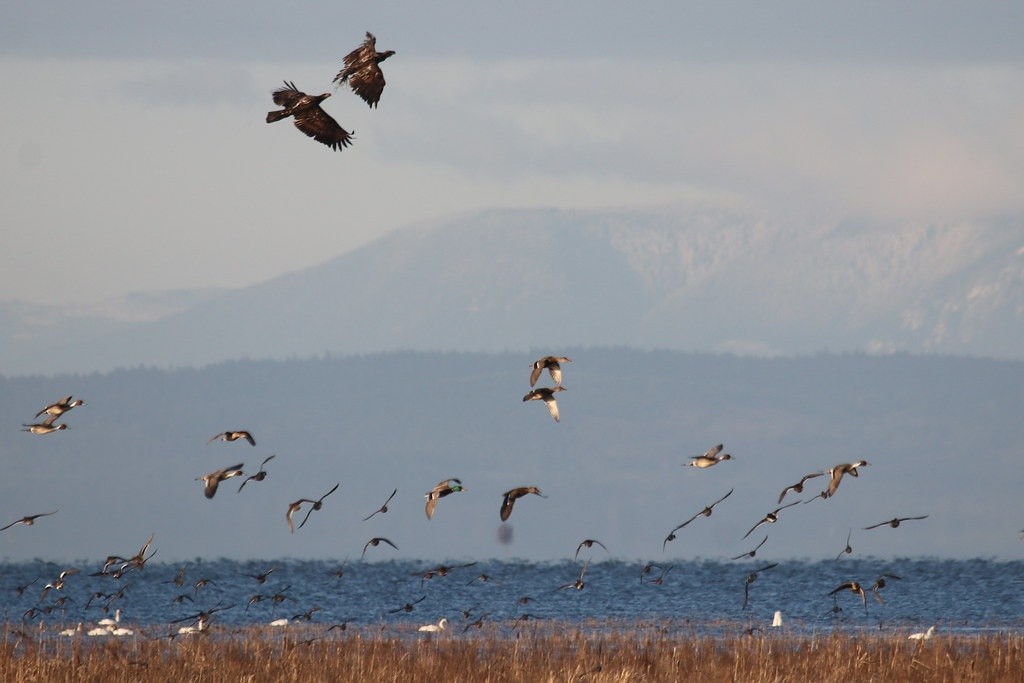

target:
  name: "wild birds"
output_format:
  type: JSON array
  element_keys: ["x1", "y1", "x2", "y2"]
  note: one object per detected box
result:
[
  {"x1": 194, "y1": 463, "x2": 244, "y2": 499},
  {"x1": 0, "y1": 512, "x2": 674, "y2": 636},
  {"x1": 286, "y1": 499, "x2": 317, "y2": 535},
  {"x1": 237, "y1": 455, "x2": 275, "y2": 493},
  {"x1": 425, "y1": 356, "x2": 571, "y2": 519},
  {"x1": 206, "y1": 431, "x2": 256, "y2": 447},
  {"x1": 827, "y1": 461, "x2": 872, "y2": 498},
  {"x1": 19, "y1": 416, "x2": 72, "y2": 435},
  {"x1": 733, "y1": 500, "x2": 927, "y2": 637},
  {"x1": 804, "y1": 490, "x2": 828, "y2": 503},
  {"x1": 909, "y1": 626, "x2": 938, "y2": 643},
  {"x1": 662, "y1": 516, "x2": 699, "y2": 553},
  {"x1": 778, "y1": 473, "x2": 826, "y2": 504},
  {"x1": 331, "y1": 30, "x2": 396, "y2": 109},
  {"x1": 34, "y1": 397, "x2": 87, "y2": 418},
  {"x1": 266, "y1": 80, "x2": 356, "y2": 152},
  {"x1": 677, "y1": 444, "x2": 735, "y2": 467},
  {"x1": 693, "y1": 488, "x2": 734, "y2": 517},
  {"x1": 500, "y1": 486, "x2": 550, "y2": 522},
  {"x1": 296, "y1": 483, "x2": 340, "y2": 530},
  {"x1": 361, "y1": 488, "x2": 397, "y2": 522}
]
